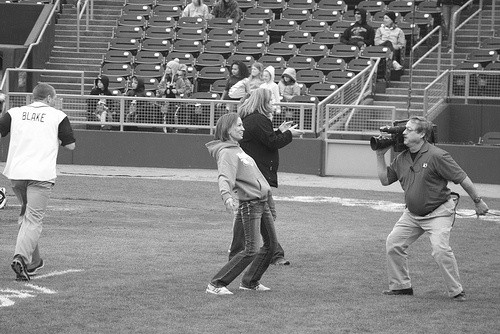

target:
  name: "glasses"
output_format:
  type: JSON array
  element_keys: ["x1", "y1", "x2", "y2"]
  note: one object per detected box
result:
[
  {"x1": 404, "y1": 127, "x2": 421, "y2": 133},
  {"x1": 354, "y1": 12, "x2": 361, "y2": 15}
]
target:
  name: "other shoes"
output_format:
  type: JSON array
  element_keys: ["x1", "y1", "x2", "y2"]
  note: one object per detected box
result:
[
  {"x1": 393, "y1": 60, "x2": 403, "y2": 71},
  {"x1": 382, "y1": 288, "x2": 415, "y2": 296},
  {"x1": 11, "y1": 257, "x2": 31, "y2": 281},
  {"x1": 453, "y1": 289, "x2": 466, "y2": 302},
  {"x1": 27, "y1": 257, "x2": 45, "y2": 275},
  {"x1": 0, "y1": 187, "x2": 8, "y2": 209},
  {"x1": 271, "y1": 256, "x2": 290, "y2": 266},
  {"x1": 238, "y1": 282, "x2": 270, "y2": 291},
  {"x1": 205, "y1": 283, "x2": 233, "y2": 295}
]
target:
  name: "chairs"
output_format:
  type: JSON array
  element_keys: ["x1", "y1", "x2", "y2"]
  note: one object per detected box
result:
[{"x1": 101, "y1": 1, "x2": 500, "y2": 123}]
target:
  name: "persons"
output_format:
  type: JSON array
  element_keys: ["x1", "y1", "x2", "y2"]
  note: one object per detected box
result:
[
  {"x1": 0, "y1": 82, "x2": 77, "y2": 281},
  {"x1": 376, "y1": 116, "x2": 490, "y2": 303},
  {"x1": 205, "y1": 88, "x2": 305, "y2": 296},
  {"x1": 86, "y1": 0, "x2": 406, "y2": 130}
]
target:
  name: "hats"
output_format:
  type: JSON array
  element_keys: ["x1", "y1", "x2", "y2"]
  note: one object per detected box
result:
[{"x1": 385, "y1": 11, "x2": 396, "y2": 22}]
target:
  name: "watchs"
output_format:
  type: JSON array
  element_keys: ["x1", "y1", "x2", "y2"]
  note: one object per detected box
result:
[{"x1": 474, "y1": 196, "x2": 483, "y2": 203}]
